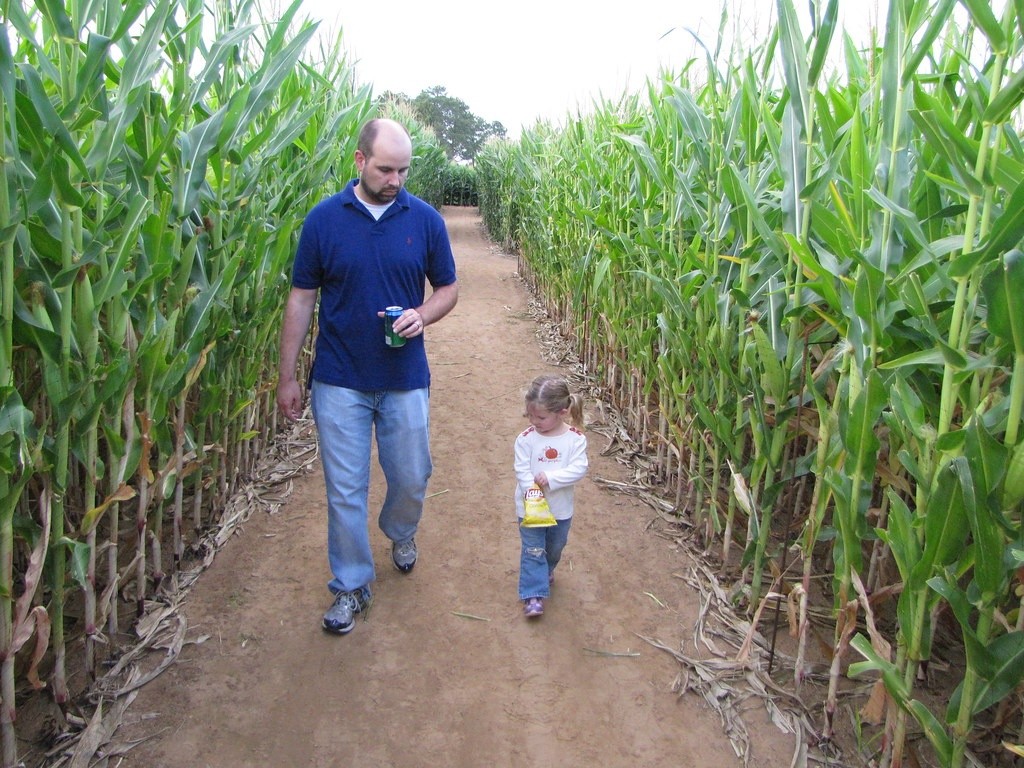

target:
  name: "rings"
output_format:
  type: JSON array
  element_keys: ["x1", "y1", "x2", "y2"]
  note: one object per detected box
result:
[{"x1": 414, "y1": 322, "x2": 421, "y2": 328}]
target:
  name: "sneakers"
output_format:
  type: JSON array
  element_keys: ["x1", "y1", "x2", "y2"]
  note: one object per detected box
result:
[
  {"x1": 523, "y1": 597, "x2": 544, "y2": 616},
  {"x1": 322, "y1": 589, "x2": 367, "y2": 632},
  {"x1": 390, "y1": 537, "x2": 418, "y2": 572},
  {"x1": 549, "y1": 573, "x2": 555, "y2": 586}
]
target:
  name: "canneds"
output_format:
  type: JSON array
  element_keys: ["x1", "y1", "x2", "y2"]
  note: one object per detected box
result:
[{"x1": 384, "y1": 306, "x2": 406, "y2": 347}]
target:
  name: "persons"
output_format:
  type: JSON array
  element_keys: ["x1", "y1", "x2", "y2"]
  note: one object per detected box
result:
[
  {"x1": 514, "y1": 374, "x2": 590, "y2": 619},
  {"x1": 275, "y1": 118, "x2": 460, "y2": 632}
]
